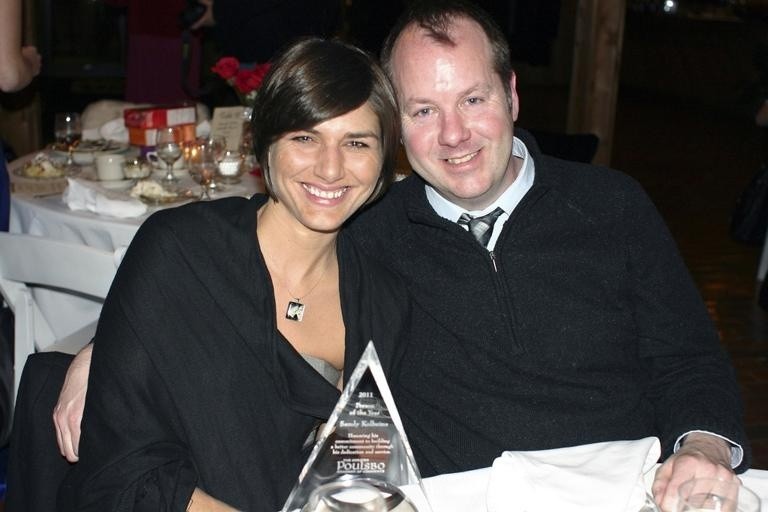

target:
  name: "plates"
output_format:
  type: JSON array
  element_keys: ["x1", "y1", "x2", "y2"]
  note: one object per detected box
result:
[
  {"x1": 134, "y1": 185, "x2": 191, "y2": 204},
  {"x1": 51, "y1": 139, "x2": 130, "y2": 156},
  {"x1": 12, "y1": 163, "x2": 83, "y2": 179}
]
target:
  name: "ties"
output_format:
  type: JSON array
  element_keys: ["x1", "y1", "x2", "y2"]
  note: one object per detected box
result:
[{"x1": 458, "y1": 206, "x2": 504, "y2": 249}]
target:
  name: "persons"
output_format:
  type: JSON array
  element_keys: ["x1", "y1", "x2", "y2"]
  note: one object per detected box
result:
[
  {"x1": 52, "y1": 1, "x2": 752, "y2": 512},
  {"x1": 59, "y1": 36, "x2": 410, "y2": 512},
  {"x1": 0, "y1": 0, "x2": 43, "y2": 232}
]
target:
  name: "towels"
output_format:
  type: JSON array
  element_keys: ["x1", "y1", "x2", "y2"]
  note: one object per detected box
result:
[{"x1": 492, "y1": 432, "x2": 663, "y2": 507}]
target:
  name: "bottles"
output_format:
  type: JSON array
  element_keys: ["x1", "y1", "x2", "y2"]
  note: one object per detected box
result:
[{"x1": 122, "y1": 156, "x2": 154, "y2": 180}]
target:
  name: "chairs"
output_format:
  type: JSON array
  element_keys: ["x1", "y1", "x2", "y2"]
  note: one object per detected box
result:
[{"x1": 1, "y1": 231, "x2": 135, "y2": 406}]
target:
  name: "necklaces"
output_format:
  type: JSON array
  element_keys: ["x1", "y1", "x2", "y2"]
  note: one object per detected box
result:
[{"x1": 268, "y1": 245, "x2": 336, "y2": 322}]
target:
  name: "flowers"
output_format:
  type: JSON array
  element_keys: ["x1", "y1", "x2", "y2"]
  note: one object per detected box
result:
[{"x1": 214, "y1": 57, "x2": 275, "y2": 103}]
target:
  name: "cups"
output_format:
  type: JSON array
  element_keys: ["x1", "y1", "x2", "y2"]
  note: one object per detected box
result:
[
  {"x1": 100, "y1": 118, "x2": 130, "y2": 145},
  {"x1": 300, "y1": 480, "x2": 388, "y2": 511},
  {"x1": 91, "y1": 154, "x2": 126, "y2": 182},
  {"x1": 677, "y1": 475, "x2": 762, "y2": 512}
]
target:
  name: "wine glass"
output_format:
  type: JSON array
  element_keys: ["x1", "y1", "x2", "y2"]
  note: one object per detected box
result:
[
  {"x1": 52, "y1": 112, "x2": 83, "y2": 168},
  {"x1": 145, "y1": 127, "x2": 245, "y2": 199}
]
